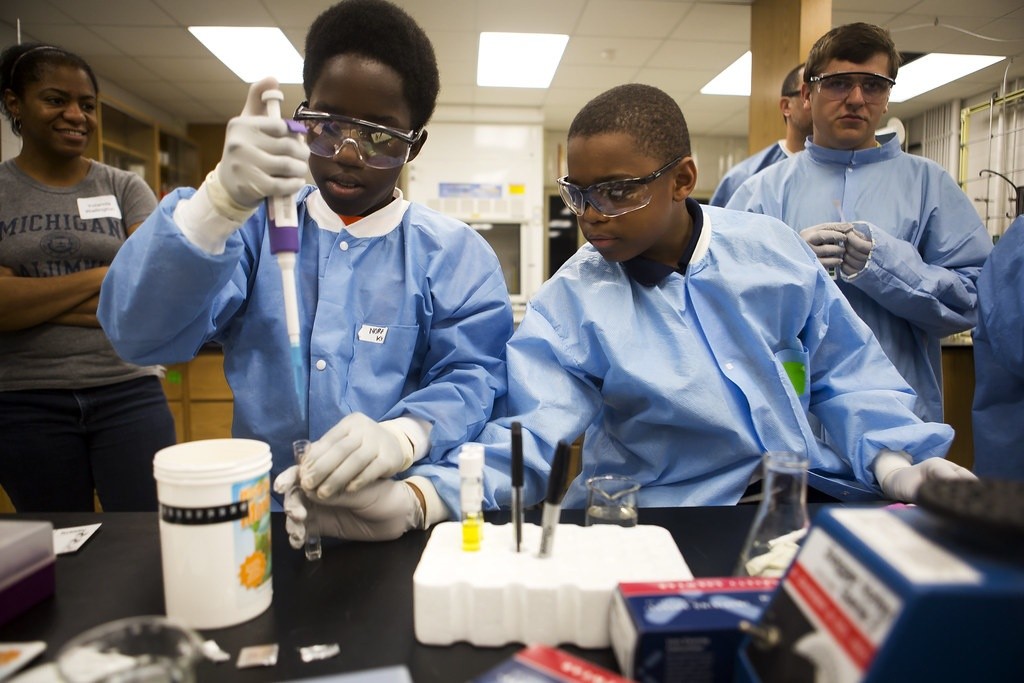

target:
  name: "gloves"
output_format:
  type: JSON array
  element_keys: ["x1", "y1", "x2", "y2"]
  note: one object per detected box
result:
[
  {"x1": 301, "y1": 410, "x2": 431, "y2": 499},
  {"x1": 801, "y1": 221, "x2": 871, "y2": 281},
  {"x1": 874, "y1": 448, "x2": 985, "y2": 515},
  {"x1": 276, "y1": 448, "x2": 429, "y2": 563},
  {"x1": 173, "y1": 74, "x2": 315, "y2": 258}
]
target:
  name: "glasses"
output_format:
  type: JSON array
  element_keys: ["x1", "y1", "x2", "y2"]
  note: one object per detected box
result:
[
  {"x1": 293, "y1": 97, "x2": 430, "y2": 170},
  {"x1": 808, "y1": 69, "x2": 897, "y2": 104},
  {"x1": 553, "y1": 156, "x2": 681, "y2": 220}
]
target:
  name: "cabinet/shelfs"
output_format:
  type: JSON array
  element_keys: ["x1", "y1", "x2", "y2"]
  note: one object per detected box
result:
[
  {"x1": 79, "y1": 96, "x2": 187, "y2": 445},
  {"x1": 184, "y1": 117, "x2": 237, "y2": 440}
]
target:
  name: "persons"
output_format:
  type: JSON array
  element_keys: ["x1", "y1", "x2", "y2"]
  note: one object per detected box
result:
[
  {"x1": 971, "y1": 214, "x2": 1023, "y2": 523},
  {"x1": 273, "y1": 83, "x2": 983, "y2": 549},
  {"x1": 0, "y1": 41, "x2": 177, "y2": 515},
  {"x1": 708, "y1": 23, "x2": 994, "y2": 424},
  {"x1": 96, "y1": 0, "x2": 514, "y2": 499}
]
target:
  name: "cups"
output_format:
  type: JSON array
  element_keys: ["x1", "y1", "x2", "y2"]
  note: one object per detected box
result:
[
  {"x1": 153, "y1": 438, "x2": 275, "y2": 630},
  {"x1": 51, "y1": 610, "x2": 207, "y2": 683}
]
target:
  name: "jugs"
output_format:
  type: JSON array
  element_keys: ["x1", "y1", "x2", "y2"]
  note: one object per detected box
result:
[{"x1": 584, "y1": 472, "x2": 641, "y2": 528}]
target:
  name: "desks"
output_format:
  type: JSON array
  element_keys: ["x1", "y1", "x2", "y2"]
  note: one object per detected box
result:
[{"x1": 0, "y1": 505, "x2": 913, "y2": 683}]
